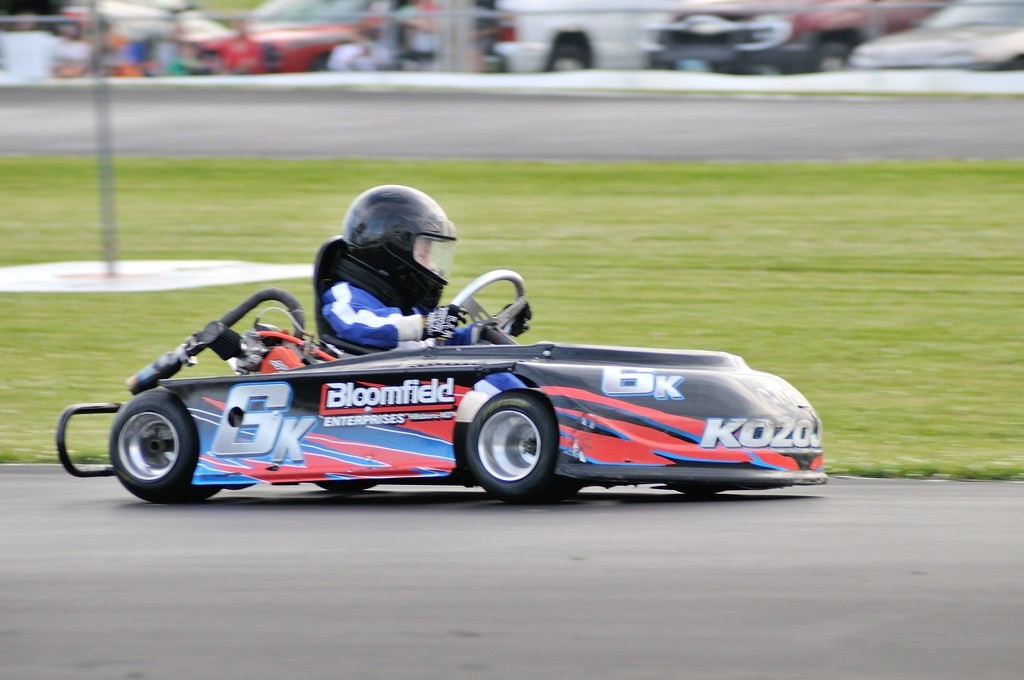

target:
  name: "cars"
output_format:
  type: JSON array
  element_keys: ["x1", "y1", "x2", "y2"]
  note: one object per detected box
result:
[{"x1": 178, "y1": 0, "x2": 1023, "y2": 75}]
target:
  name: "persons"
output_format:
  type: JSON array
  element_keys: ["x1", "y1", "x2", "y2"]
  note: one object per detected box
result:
[
  {"x1": 2, "y1": 0, "x2": 502, "y2": 79},
  {"x1": 321, "y1": 183, "x2": 533, "y2": 348}
]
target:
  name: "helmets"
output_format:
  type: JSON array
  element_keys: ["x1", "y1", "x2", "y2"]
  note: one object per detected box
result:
[{"x1": 341, "y1": 184, "x2": 455, "y2": 313}]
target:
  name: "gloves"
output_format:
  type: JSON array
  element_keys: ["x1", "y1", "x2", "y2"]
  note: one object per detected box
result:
[
  {"x1": 491, "y1": 302, "x2": 532, "y2": 338},
  {"x1": 421, "y1": 303, "x2": 475, "y2": 342}
]
